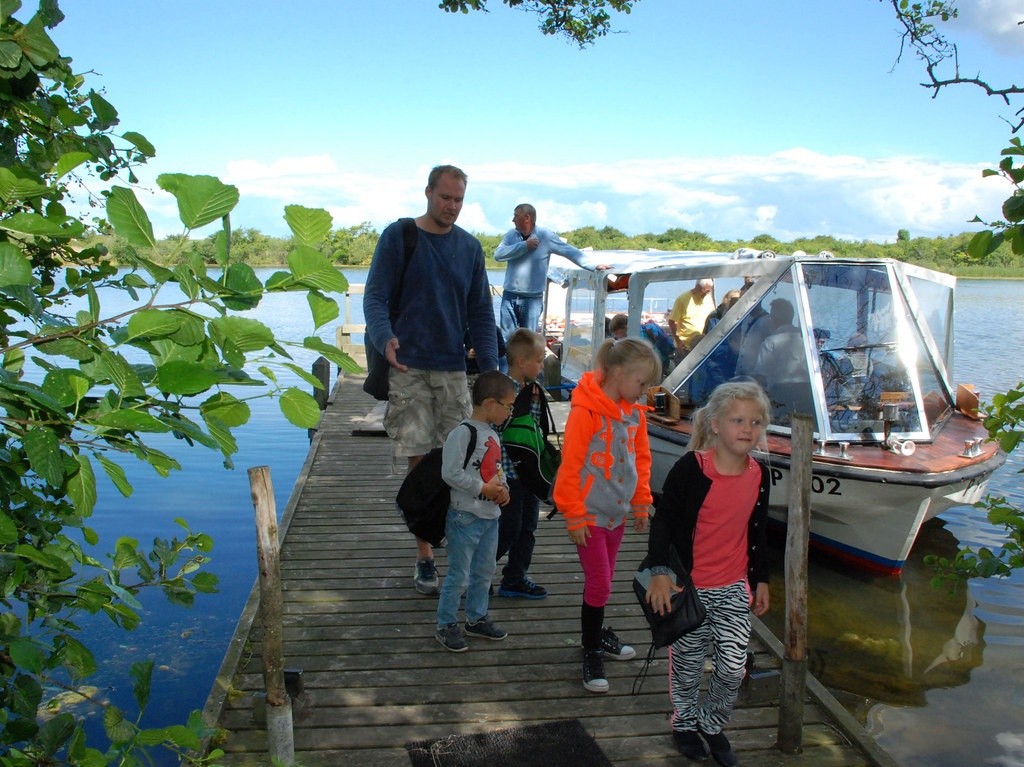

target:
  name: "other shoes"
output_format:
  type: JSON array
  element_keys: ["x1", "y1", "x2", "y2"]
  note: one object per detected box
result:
[
  {"x1": 696, "y1": 722, "x2": 738, "y2": 767},
  {"x1": 672, "y1": 729, "x2": 709, "y2": 762}
]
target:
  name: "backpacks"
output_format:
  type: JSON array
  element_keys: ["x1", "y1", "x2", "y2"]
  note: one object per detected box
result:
[
  {"x1": 505, "y1": 380, "x2": 562, "y2": 520},
  {"x1": 643, "y1": 319, "x2": 675, "y2": 359},
  {"x1": 395, "y1": 422, "x2": 478, "y2": 546}
]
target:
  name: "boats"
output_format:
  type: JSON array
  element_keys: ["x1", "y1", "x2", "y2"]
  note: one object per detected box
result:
[{"x1": 538, "y1": 246, "x2": 1012, "y2": 581}]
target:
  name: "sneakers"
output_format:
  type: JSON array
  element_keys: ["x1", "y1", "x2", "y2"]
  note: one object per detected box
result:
[
  {"x1": 498, "y1": 577, "x2": 547, "y2": 600},
  {"x1": 464, "y1": 613, "x2": 508, "y2": 640},
  {"x1": 582, "y1": 647, "x2": 610, "y2": 692},
  {"x1": 414, "y1": 557, "x2": 442, "y2": 595},
  {"x1": 435, "y1": 623, "x2": 469, "y2": 653},
  {"x1": 596, "y1": 625, "x2": 637, "y2": 661}
]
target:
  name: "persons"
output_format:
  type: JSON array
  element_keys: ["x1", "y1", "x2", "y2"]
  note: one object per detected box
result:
[
  {"x1": 494, "y1": 205, "x2": 615, "y2": 345},
  {"x1": 436, "y1": 276, "x2": 875, "y2": 692},
  {"x1": 362, "y1": 164, "x2": 501, "y2": 595},
  {"x1": 646, "y1": 382, "x2": 775, "y2": 767}
]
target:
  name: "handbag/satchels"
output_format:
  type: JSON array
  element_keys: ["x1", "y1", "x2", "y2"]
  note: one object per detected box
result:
[
  {"x1": 362, "y1": 322, "x2": 394, "y2": 401},
  {"x1": 633, "y1": 555, "x2": 707, "y2": 698}
]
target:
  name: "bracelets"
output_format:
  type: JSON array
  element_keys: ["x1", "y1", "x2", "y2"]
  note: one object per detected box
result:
[{"x1": 650, "y1": 568, "x2": 668, "y2": 576}]
